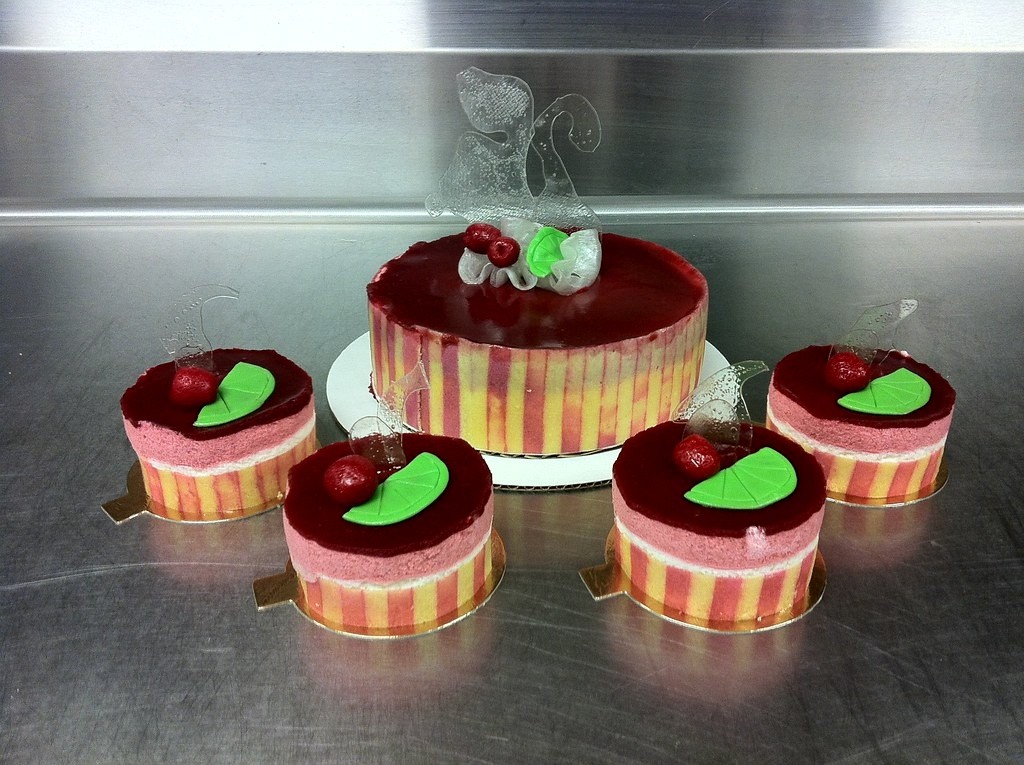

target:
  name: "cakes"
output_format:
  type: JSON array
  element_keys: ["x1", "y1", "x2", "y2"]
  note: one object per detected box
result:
[
  {"x1": 278, "y1": 361, "x2": 495, "y2": 627},
  {"x1": 364, "y1": 68, "x2": 709, "y2": 456},
  {"x1": 118, "y1": 284, "x2": 318, "y2": 514},
  {"x1": 610, "y1": 360, "x2": 827, "y2": 621},
  {"x1": 765, "y1": 298, "x2": 955, "y2": 498}
]
